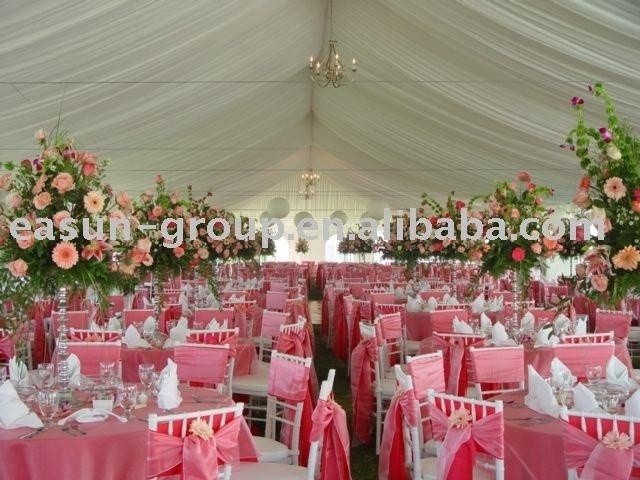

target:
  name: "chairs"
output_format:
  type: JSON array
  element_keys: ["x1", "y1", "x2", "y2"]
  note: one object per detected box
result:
[{"x1": 0, "y1": 260, "x2": 640, "y2": 480}]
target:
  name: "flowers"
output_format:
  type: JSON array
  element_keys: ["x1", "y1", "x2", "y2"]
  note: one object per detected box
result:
[{"x1": 0, "y1": 80, "x2": 640, "y2": 369}]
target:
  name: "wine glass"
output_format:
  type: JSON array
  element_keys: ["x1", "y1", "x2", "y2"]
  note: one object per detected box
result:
[
  {"x1": 467, "y1": 317, "x2": 621, "y2": 416},
  {"x1": 36, "y1": 318, "x2": 204, "y2": 428}
]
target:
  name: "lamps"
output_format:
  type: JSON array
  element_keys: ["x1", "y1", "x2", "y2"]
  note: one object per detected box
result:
[{"x1": 308, "y1": 0, "x2": 357, "y2": 88}]
